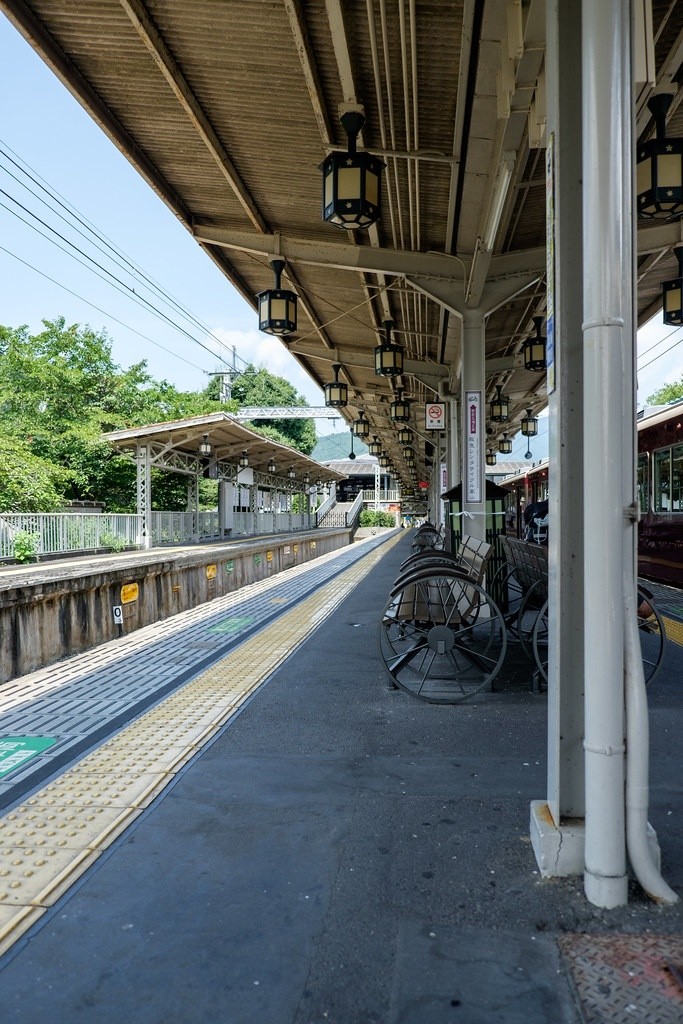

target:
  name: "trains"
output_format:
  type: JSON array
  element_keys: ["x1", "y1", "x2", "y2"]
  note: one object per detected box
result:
[{"x1": 496, "y1": 397, "x2": 682, "y2": 591}]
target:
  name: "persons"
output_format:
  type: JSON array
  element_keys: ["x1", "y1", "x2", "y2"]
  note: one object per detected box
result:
[
  {"x1": 411, "y1": 514, "x2": 416, "y2": 528},
  {"x1": 405, "y1": 513, "x2": 409, "y2": 527}
]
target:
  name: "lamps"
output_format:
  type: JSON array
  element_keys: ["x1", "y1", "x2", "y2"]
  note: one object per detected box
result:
[
  {"x1": 368, "y1": 436, "x2": 382, "y2": 457},
  {"x1": 375, "y1": 320, "x2": 403, "y2": 380},
  {"x1": 239, "y1": 451, "x2": 249, "y2": 469},
  {"x1": 199, "y1": 435, "x2": 212, "y2": 456},
  {"x1": 489, "y1": 385, "x2": 509, "y2": 425},
  {"x1": 267, "y1": 459, "x2": 419, "y2": 489},
  {"x1": 403, "y1": 447, "x2": 415, "y2": 460},
  {"x1": 397, "y1": 426, "x2": 415, "y2": 445},
  {"x1": 522, "y1": 316, "x2": 547, "y2": 373},
  {"x1": 636, "y1": 93, "x2": 683, "y2": 221},
  {"x1": 659, "y1": 245, "x2": 683, "y2": 327},
  {"x1": 254, "y1": 260, "x2": 299, "y2": 337},
  {"x1": 486, "y1": 449, "x2": 496, "y2": 466},
  {"x1": 390, "y1": 387, "x2": 409, "y2": 423},
  {"x1": 317, "y1": 112, "x2": 387, "y2": 231},
  {"x1": 520, "y1": 409, "x2": 538, "y2": 437},
  {"x1": 324, "y1": 364, "x2": 349, "y2": 408},
  {"x1": 378, "y1": 451, "x2": 391, "y2": 468},
  {"x1": 497, "y1": 433, "x2": 512, "y2": 454},
  {"x1": 353, "y1": 411, "x2": 370, "y2": 438}
]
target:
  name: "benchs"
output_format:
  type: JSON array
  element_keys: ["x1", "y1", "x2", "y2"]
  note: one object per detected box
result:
[
  {"x1": 417, "y1": 522, "x2": 437, "y2": 531},
  {"x1": 497, "y1": 534, "x2": 659, "y2": 636},
  {"x1": 413, "y1": 528, "x2": 453, "y2": 567},
  {"x1": 411, "y1": 522, "x2": 443, "y2": 551},
  {"x1": 382, "y1": 535, "x2": 495, "y2": 693}
]
